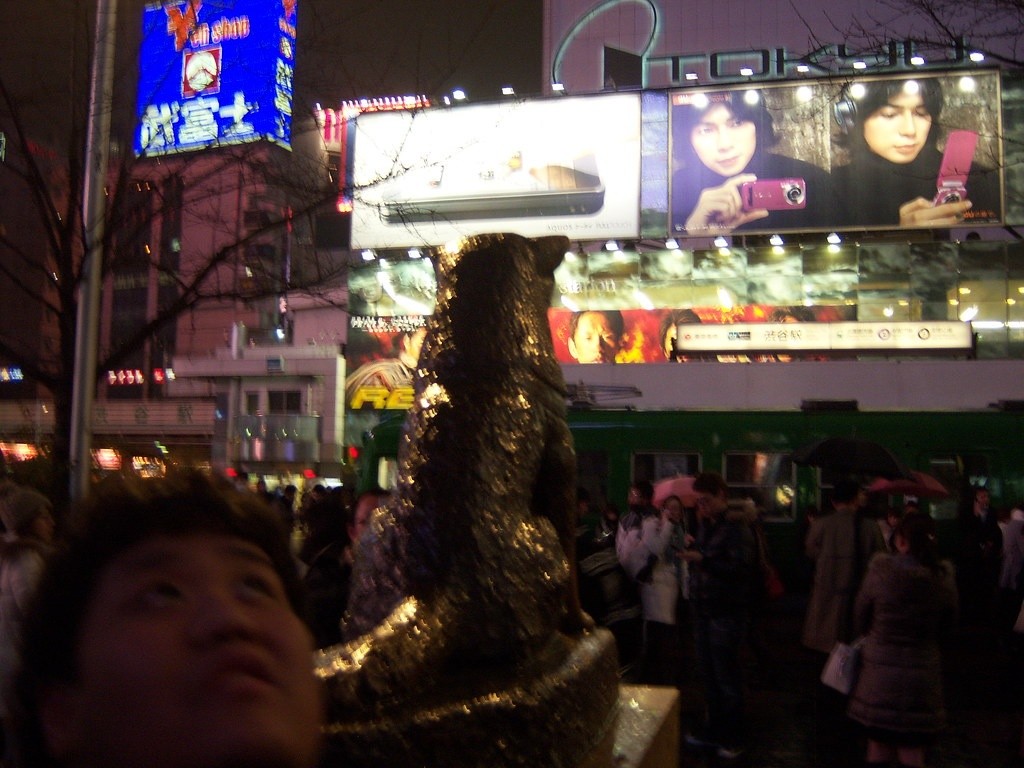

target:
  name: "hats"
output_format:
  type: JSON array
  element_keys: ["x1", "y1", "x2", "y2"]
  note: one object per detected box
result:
[{"x1": 1, "y1": 480, "x2": 52, "y2": 531}]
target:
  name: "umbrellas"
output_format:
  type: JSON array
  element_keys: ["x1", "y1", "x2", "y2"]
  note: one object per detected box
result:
[
  {"x1": 650, "y1": 476, "x2": 707, "y2": 543},
  {"x1": 791, "y1": 437, "x2": 951, "y2": 525}
]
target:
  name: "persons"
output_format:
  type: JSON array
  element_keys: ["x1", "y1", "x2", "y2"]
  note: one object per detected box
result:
[
  {"x1": 20, "y1": 473, "x2": 321, "y2": 768},
  {"x1": 356, "y1": 310, "x2": 858, "y2": 363},
  {"x1": 229, "y1": 472, "x2": 1024, "y2": 766},
  {"x1": 669, "y1": 75, "x2": 1002, "y2": 228},
  {"x1": 0, "y1": 484, "x2": 55, "y2": 688}
]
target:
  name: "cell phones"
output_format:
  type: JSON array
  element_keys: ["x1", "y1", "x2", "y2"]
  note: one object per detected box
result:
[
  {"x1": 741, "y1": 178, "x2": 806, "y2": 211},
  {"x1": 932, "y1": 130, "x2": 979, "y2": 208}
]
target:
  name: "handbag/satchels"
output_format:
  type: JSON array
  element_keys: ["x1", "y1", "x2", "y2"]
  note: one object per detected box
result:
[{"x1": 821, "y1": 637, "x2": 871, "y2": 698}]
